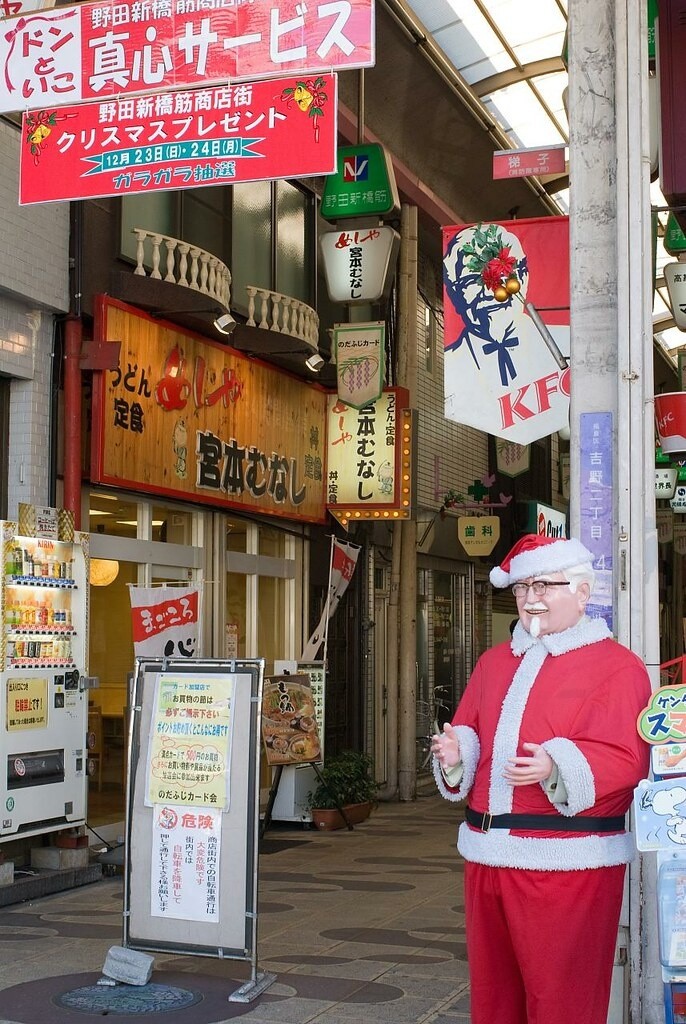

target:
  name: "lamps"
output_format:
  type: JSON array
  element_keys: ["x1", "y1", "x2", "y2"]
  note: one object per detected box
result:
[
  {"x1": 248, "y1": 348, "x2": 325, "y2": 375},
  {"x1": 148, "y1": 307, "x2": 236, "y2": 340},
  {"x1": 90, "y1": 524, "x2": 119, "y2": 587}
]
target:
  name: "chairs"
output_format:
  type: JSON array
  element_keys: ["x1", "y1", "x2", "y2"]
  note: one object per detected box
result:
[{"x1": 86, "y1": 706, "x2": 104, "y2": 793}]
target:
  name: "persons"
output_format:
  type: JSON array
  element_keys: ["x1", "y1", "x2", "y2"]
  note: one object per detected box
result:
[{"x1": 430, "y1": 535, "x2": 651, "y2": 1024}]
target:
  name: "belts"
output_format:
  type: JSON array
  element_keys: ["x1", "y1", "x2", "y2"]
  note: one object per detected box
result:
[{"x1": 465, "y1": 805, "x2": 626, "y2": 834}]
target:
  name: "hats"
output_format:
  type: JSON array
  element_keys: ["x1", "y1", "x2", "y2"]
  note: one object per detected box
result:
[{"x1": 489, "y1": 533, "x2": 596, "y2": 589}]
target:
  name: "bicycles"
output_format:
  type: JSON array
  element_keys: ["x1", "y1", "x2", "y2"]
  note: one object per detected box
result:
[{"x1": 416, "y1": 684, "x2": 454, "y2": 797}]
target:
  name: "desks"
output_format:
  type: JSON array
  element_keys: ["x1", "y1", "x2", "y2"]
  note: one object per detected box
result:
[{"x1": 102, "y1": 706, "x2": 127, "y2": 795}]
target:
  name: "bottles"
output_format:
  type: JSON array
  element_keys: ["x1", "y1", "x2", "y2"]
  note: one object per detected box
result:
[
  {"x1": 12, "y1": 540, "x2": 71, "y2": 580},
  {"x1": 6, "y1": 600, "x2": 71, "y2": 627}
]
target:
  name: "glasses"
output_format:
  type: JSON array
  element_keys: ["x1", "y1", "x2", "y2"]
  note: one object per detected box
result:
[{"x1": 510, "y1": 580, "x2": 570, "y2": 597}]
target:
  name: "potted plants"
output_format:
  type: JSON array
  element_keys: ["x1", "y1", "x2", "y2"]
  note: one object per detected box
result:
[{"x1": 294, "y1": 748, "x2": 387, "y2": 831}]
